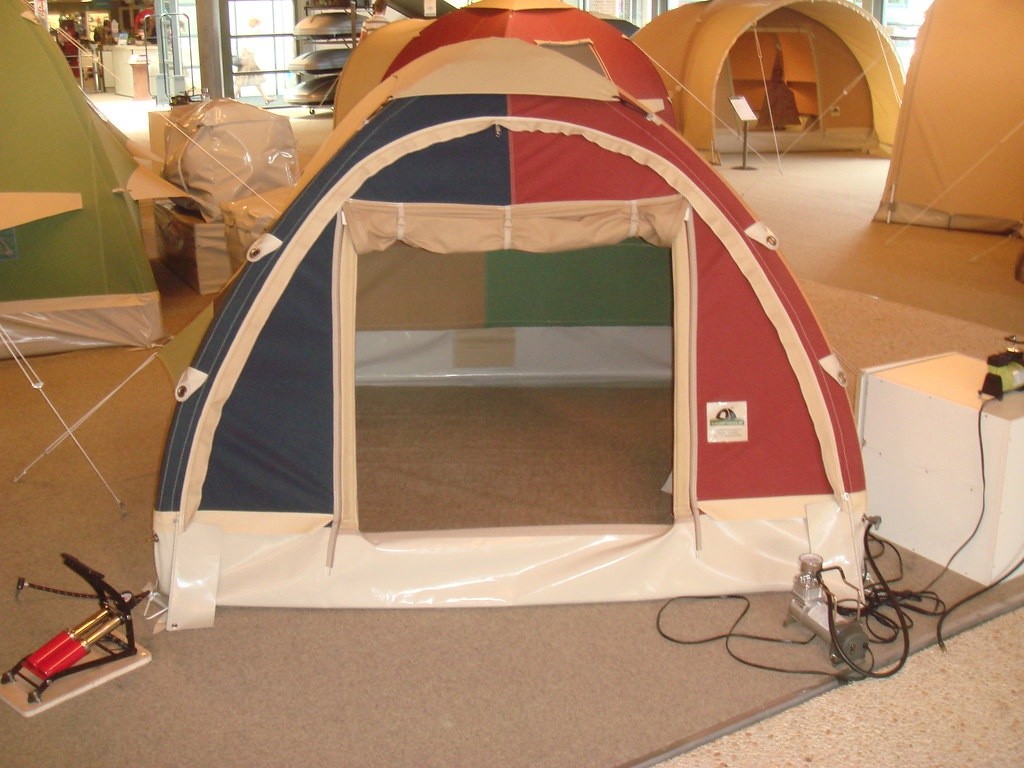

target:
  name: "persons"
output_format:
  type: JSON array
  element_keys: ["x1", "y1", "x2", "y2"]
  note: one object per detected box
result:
[
  {"x1": 94, "y1": 20, "x2": 112, "y2": 44},
  {"x1": 234, "y1": 19, "x2": 279, "y2": 105},
  {"x1": 359, "y1": 0, "x2": 392, "y2": 42}
]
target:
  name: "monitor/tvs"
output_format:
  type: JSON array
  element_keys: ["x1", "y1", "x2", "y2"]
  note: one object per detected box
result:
[{"x1": 117, "y1": 33, "x2": 128, "y2": 47}]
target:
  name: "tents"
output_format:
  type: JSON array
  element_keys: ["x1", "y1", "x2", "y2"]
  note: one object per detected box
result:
[
  {"x1": 0, "y1": 0, "x2": 165, "y2": 517},
  {"x1": 867, "y1": 1, "x2": 1024, "y2": 237},
  {"x1": 313, "y1": 0, "x2": 776, "y2": 172},
  {"x1": 627, "y1": 1, "x2": 905, "y2": 168},
  {"x1": 12, "y1": 32, "x2": 870, "y2": 629}
]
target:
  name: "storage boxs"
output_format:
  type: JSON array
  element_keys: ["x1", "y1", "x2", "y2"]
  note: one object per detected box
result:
[
  {"x1": 154, "y1": 198, "x2": 235, "y2": 296},
  {"x1": 852, "y1": 352, "x2": 1024, "y2": 587}
]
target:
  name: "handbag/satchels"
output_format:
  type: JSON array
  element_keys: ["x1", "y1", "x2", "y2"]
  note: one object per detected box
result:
[{"x1": 232, "y1": 56, "x2": 241, "y2": 66}]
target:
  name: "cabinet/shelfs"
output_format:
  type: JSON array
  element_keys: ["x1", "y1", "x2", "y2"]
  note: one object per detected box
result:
[
  {"x1": 77, "y1": 43, "x2": 162, "y2": 98},
  {"x1": 299, "y1": 2, "x2": 360, "y2": 115}
]
